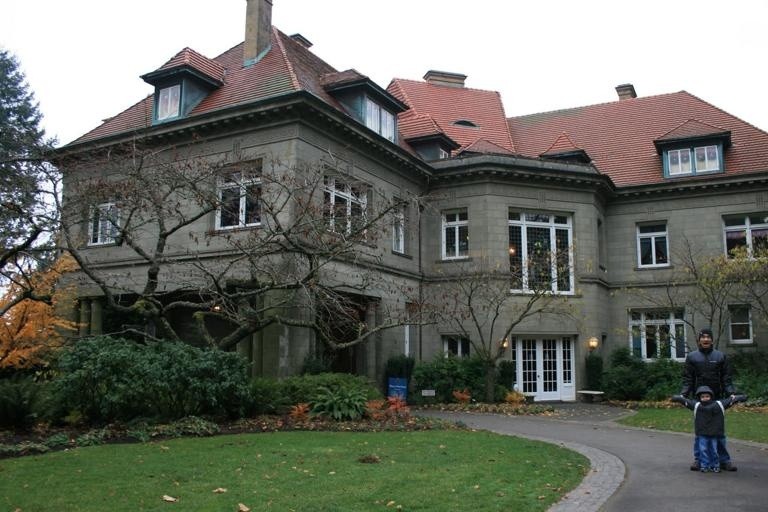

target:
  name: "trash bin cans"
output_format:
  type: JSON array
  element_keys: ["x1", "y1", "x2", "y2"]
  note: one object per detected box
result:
[{"x1": 387, "y1": 377, "x2": 407, "y2": 400}]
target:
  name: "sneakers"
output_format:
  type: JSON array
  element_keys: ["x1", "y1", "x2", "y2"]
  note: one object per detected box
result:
[
  {"x1": 719, "y1": 463, "x2": 736, "y2": 472},
  {"x1": 713, "y1": 462, "x2": 721, "y2": 473},
  {"x1": 690, "y1": 463, "x2": 700, "y2": 471},
  {"x1": 700, "y1": 466, "x2": 711, "y2": 472}
]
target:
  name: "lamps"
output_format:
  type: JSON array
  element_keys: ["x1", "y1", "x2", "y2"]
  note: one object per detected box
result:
[
  {"x1": 589, "y1": 337, "x2": 600, "y2": 353},
  {"x1": 499, "y1": 338, "x2": 508, "y2": 354}
]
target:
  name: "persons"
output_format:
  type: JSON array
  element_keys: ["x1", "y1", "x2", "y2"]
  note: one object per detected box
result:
[
  {"x1": 666, "y1": 384, "x2": 750, "y2": 473},
  {"x1": 678, "y1": 327, "x2": 739, "y2": 472}
]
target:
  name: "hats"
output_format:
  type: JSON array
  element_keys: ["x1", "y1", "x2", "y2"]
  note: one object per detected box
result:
[{"x1": 698, "y1": 329, "x2": 713, "y2": 342}]
